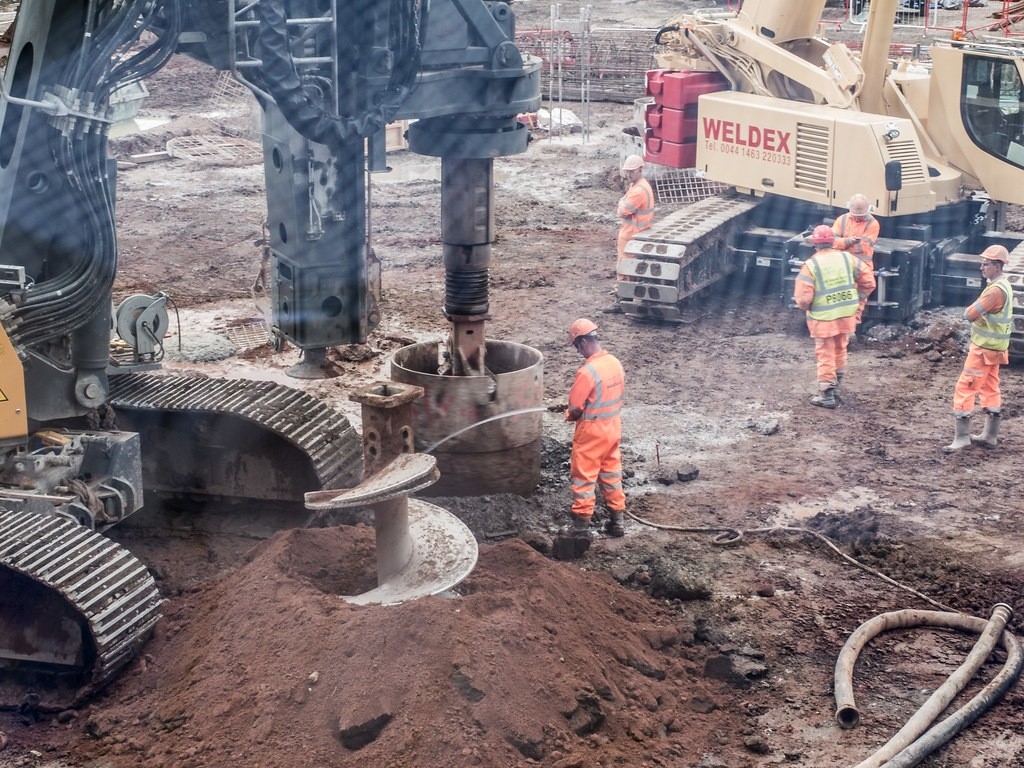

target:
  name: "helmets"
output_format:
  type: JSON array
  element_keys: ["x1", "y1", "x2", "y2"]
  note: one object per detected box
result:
[
  {"x1": 566, "y1": 318, "x2": 599, "y2": 347},
  {"x1": 848, "y1": 194, "x2": 870, "y2": 217},
  {"x1": 622, "y1": 154, "x2": 645, "y2": 170},
  {"x1": 979, "y1": 245, "x2": 1009, "y2": 264},
  {"x1": 812, "y1": 225, "x2": 835, "y2": 244}
]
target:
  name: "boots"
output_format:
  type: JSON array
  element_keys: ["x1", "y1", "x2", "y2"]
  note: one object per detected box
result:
[
  {"x1": 941, "y1": 413, "x2": 973, "y2": 453},
  {"x1": 969, "y1": 413, "x2": 1003, "y2": 448}
]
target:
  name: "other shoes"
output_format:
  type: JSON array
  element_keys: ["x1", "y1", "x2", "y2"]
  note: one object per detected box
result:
[
  {"x1": 603, "y1": 518, "x2": 625, "y2": 536},
  {"x1": 557, "y1": 524, "x2": 594, "y2": 542},
  {"x1": 602, "y1": 292, "x2": 633, "y2": 314},
  {"x1": 809, "y1": 395, "x2": 836, "y2": 409}
]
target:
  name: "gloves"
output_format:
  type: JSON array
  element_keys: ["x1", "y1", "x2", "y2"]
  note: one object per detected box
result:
[{"x1": 844, "y1": 236, "x2": 861, "y2": 247}]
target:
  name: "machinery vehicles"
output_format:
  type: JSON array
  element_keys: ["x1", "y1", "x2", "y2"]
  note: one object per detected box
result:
[
  {"x1": 613, "y1": 0, "x2": 1024, "y2": 369},
  {"x1": 0, "y1": 0, "x2": 557, "y2": 718}
]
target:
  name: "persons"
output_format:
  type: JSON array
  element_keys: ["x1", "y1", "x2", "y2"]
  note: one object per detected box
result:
[
  {"x1": 602, "y1": 155, "x2": 654, "y2": 314},
  {"x1": 831, "y1": 195, "x2": 881, "y2": 344},
  {"x1": 565, "y1": 318, "x2": 627, "y2": 537},
  {"x1": 941, "y1": 244, "x2": 1012, "y2": 453},
  {"x1": 794, "y1": 225, "x2": 875, "y2": 408}
]
